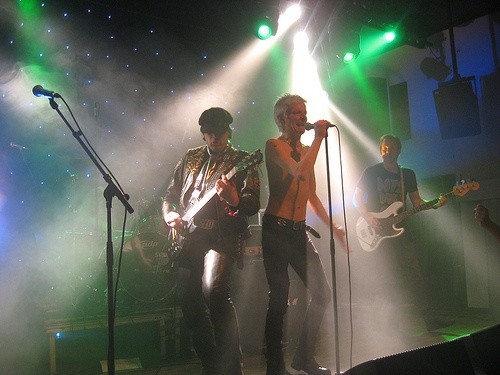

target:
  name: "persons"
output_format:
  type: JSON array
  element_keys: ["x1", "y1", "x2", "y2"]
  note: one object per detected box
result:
[
  {"x1": 355, "y1": 135, "x2": 448, "y2": 301},
  {"x1": 160, "y1": 107, "x2": 260, "y2": 374},
  {"x1": 472, "y1": 204, "x2": 499, "y2": 245},
  {"x1": 261, "y1": 93, "x2": 352, "y2": 375}
]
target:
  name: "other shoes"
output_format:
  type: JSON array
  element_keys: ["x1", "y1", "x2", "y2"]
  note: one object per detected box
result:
[{"x1": 426, "y1": 317, "x2": 457, "y2": 333}]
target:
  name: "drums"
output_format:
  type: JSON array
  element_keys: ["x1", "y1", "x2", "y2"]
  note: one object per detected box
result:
[
  {"x1": 66, "y1": 232, "x2": 104, "y2": 272},
  {"x1": 115, "y1": 232, "x2": 180, "y2": 304}
]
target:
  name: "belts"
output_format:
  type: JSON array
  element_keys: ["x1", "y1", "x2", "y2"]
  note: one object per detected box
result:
[
  {"x1": 264, "y1": 214, "x2": 320, "y2": 238},
  {"x1": 194, "y1": 219, "x2": 218, "y2": 230}
]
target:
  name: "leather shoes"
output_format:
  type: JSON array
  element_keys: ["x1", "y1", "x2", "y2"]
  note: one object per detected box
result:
[
  {"x1": 265, "y1": 364, "x2": 290, "y2": 375},
  {"x1": 290, "y1": 352, "x2": 331, "y2": 375}
]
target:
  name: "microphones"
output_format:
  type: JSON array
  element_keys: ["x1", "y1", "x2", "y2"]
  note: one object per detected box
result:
[
  {"x1": 304, "y1": 121, "x2": 336, "y2": 131},
  {"x1": 32, "y1": 84, "x2": 62, "y2": 99}
]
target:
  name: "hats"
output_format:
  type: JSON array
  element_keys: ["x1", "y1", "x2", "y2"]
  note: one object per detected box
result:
[{"x1": 199, "y1": 107, "x2": 233, "y2": 133}]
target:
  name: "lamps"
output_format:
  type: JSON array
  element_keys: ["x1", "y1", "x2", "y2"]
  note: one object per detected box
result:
[
  {"x1": 419, "y1": 56, "x2": 450, "y2": 82},
  {"x1": 239, "y1": 0, "x2": 279, "y2": 40},
  {"x1": 327, "y1": 18, "x2": 362, "y2": 65}
]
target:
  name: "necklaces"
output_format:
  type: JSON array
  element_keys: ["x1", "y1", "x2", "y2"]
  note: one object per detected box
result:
[{"x1": 279, "y1": 136, "x2": 295, "y2": 149}]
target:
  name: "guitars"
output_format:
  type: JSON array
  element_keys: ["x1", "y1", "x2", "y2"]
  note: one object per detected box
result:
[
  {"x1": 165, "y1": 150, "x2": 264, "y2": 261},
  {"x1": 357, "y1": 179, "x2": 480, "y2": 253}
]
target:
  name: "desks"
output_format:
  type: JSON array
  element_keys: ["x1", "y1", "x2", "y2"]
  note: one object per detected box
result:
[{"x1": 42, "y1": 304, "x2": 185, "y2": 375}]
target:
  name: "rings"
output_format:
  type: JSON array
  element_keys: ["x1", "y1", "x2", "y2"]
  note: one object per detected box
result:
[{"x1": 476, "y1": 213, "x2": 480, "y2": 216}]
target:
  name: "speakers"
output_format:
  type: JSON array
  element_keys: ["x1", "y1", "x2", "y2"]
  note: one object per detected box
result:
[
  {"x1": 465, "y1": 323, "x2": 500, "y2": 375},
  {"x1": 233, "y1": 241, "x2": 301, "y2": 351},
  {"x1": 337, "y1": 332, "x2": 483, "y2": 375}
]
